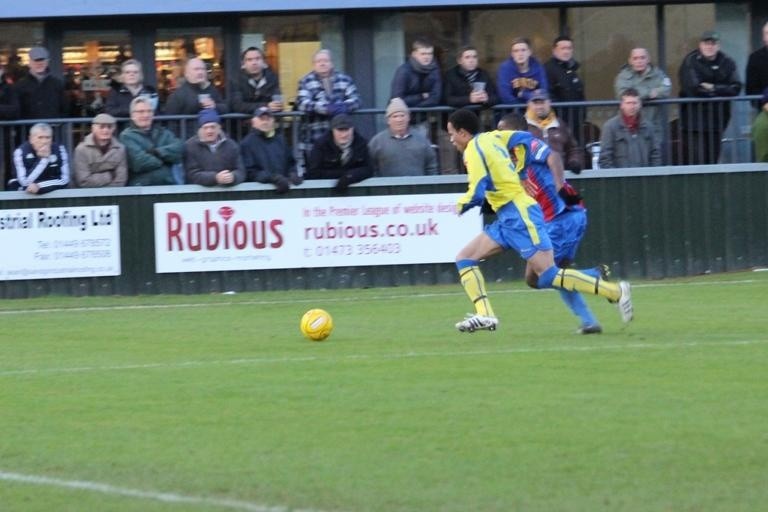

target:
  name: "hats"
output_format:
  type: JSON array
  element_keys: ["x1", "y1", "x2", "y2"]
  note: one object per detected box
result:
[
  {"x1": 385, "y1": 97, "x2": 410, "y2": 117},
  {"x1": 700, "y1": 30, "x2": 721, "y2": 41},
  {"x1": 28, "y1": 46, "x2": 50, "y2": 60},
  {"x1": 196, "y1": 108, "x2": 221, "y2": 129},
  {"x1": 254, "y1": 106, "x2": 273, "y2": 117},
  {"x1": 330, "y1": 113, "x2": 355, "y2": 129},
  {"x1": 530, "y1": 88, "x2": 549, "y2": 101}
]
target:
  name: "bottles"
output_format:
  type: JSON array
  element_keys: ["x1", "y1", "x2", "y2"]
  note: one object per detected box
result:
[{"x1": 589, "y1": 145, "x2": 601, "y2": 169}]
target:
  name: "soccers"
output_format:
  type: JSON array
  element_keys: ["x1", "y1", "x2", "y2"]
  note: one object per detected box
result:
[{"x1": 300, "y1": 309, "x2": 332, "y2": 340}]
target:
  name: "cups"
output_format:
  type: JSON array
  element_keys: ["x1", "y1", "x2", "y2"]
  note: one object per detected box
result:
[
  {"x1": 471, "y1": 79, "x2": 487, "y2": 92},
  {"x1": 197, "y1": 92, "x2": 211, "y2": 109},
  {"x1": 273, "y1": 94, "x2": 284, "y2": 111}
]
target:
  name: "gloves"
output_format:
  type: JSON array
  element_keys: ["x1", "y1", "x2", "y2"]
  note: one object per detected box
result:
[
  {"x1": 334, "y1": 174, "x2": 350, "y2": 195},
  {"x1": 275, "y1": 179, "x2": 289, "y2": 195},
  {"x1": 567, "y1": 159, "x2": 582, "y2": 175},
  {"x1": 290, "y1": 174, "x2": 303, "y2": 186},
  {"x1": 556, "y1": 181, "x2": 584, "y2": 210}
]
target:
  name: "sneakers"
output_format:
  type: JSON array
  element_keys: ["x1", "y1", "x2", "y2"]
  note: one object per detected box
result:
[
  {"x1": 572, "y1": 319, "x2": 602, "y2": 335},
  {"x1": 596, "y1": 263, "x2": 621, "y2": 304},
  {"x1": 616, "y1": 279, "x2": 633, "y2": 323},
  {"x1": 454, "y1": 314, "x2": 498, "y2": 334}
]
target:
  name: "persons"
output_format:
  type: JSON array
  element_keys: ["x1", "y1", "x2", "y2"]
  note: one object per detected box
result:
[
  {"x1": 598, "y1": 88, "x2": 661, "y2": 170},
  {"x1": 437, "y1": 46, "x2": 495, "y2": 175},
  {"x1": 366, "y1": 97, "x2": 439, "y2": 177},
  {"x1": 495, "y1": 38, "x2": 552, "y2": 117},
  {"x1": 446, "y1": 109, "x2": 634, "y2": 334},
  {"x1": 522, "y1": 89, "x2": 583, "y2": 174},
  {"x1": 1, "y1": 46, "x2": 375, "y2": 194},
  {"x1": 497, "y1": 112, "x2": 614, "y2": 334},
  {"x1": 745, "y1": 21, "x2": 768, "y2": 162},
  {"x1": 392, "y1": 39, "x2": 444, "y2": 144},
  {"x1": 543, "y1": 37, "x2": 588, "y2": 145},
  {"x1": 614, "y1": 48, "x2": 673, "y2": 165},
  {"x1": 678, "y1": 31, "x2": 742, "y2": 165}
]
